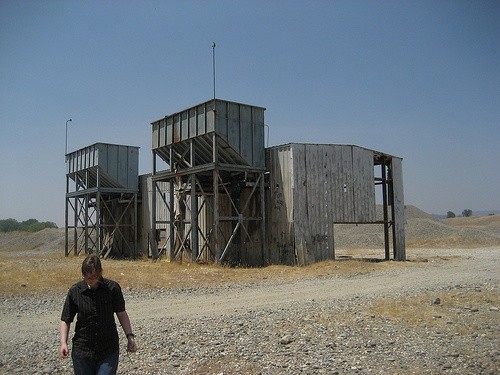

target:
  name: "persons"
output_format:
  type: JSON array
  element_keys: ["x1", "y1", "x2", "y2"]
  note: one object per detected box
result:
[{"x1": 59, "y1": 256, "x2": 138, "y2": 375}]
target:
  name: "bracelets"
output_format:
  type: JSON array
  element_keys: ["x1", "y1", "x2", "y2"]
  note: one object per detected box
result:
[{"x1": 125, "y1": 333, "x2": 134, "y2": 338}]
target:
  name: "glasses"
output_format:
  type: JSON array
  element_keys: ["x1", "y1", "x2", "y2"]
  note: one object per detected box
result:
[{"x1": 82, "y1": 273, "x2": 99, "y2": 281}]
